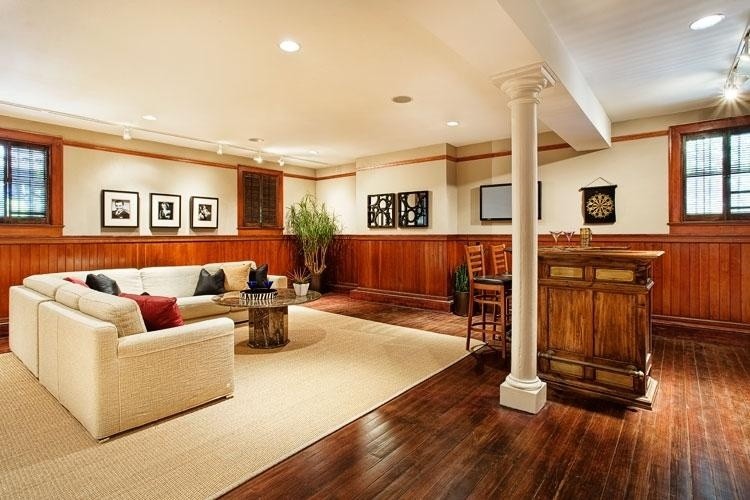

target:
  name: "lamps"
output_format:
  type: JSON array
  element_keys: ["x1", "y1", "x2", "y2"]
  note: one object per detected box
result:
[
  {"x1": 121, "y1": 126, "x2": 133, "y2": 141},
  {"x1": 720, "y1": 22, "x2": 750, "y2": 102},
  {"x1": 213, "y1": 143, "x2": 285, "y2": 167}
]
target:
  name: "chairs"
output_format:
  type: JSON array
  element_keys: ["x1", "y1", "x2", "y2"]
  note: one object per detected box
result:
[
  {"x1": 492, "y1": 245, "x2": 512, "y2": 340},
  {"x1": 463, "y1": 243, "x2": 511, "y2": 358}
]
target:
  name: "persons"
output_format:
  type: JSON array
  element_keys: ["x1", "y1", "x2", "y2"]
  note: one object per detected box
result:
[
  {"x1": 112, "y1": 200, "x2": 129, "y2": 218},
  {"x1": 159, "y1": 202, "x2": 172, "y2": 219},
  {"x1": 199, "y1": 209, "x2": 205, "y2": 221},
  {"x1": 200, "y1": 205, "x2": 211, "y2": 219}
]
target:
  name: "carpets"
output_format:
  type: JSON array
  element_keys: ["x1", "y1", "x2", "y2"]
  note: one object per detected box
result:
[{"x1": 1, "y1": 305, "x2": 486, "y2": 500}]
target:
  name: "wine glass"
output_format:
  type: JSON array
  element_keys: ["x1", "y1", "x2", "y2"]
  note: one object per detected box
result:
[
  {"x1": 563, "y1": 231, "x2": 576, "y2": 248},
  {"x1": 548, "y1": 230, "x2": 563, "y2": 250}
]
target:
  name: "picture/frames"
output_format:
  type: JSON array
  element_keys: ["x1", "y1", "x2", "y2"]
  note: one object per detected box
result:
[
  {"x1": 189, "y1": 195, "x2": 219, "y2": 230},
  {"x1": 148, "y1": 192, "x2": 182, "y2": 229},
  {"x1": 101, "y1": 189, "x2": 140, "y2": 229}
]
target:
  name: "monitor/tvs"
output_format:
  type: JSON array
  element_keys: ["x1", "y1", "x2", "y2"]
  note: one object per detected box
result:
[{"x1": 480, "y1": 180, "x2": 542, "y2": 222}]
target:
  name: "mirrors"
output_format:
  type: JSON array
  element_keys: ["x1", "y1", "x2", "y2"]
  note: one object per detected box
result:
[
  {"x1": 366, "y1": 192, "x2": 396, "y2": 228},
  {"x1": 397, "y1": 190, "x2": 430, "y2": 228}
]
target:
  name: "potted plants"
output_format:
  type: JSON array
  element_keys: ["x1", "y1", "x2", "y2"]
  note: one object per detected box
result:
[
  {"x1": 286, "y1": 269, "x2": 313, "y2": 296},
  {"x1": 453, "y1": 263, "x2": 476, "y2": 317}
]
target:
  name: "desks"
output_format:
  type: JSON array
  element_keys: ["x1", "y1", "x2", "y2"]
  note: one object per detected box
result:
[{"x1": 538, "y1": 246, "x2": 668, "y2": 411}]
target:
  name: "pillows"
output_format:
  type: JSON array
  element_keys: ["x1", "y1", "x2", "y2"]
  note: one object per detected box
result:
[
  {"x1": 85, "y1": 273, "x2": 122, "y2": 297},
  {"x1": 220, "y1": 263, "x2": 252, "y2": 291},
  {"x1": 192, "y1": 268, "x2": 226, "y2": 296},
  {"x1": 118, "y1": 292, "x2": 184, "y2": 332},
  {"x1": 248, "y1": 263, "x2": 268, "y2": 282},
  {"x1": 63, "y1": 276, "x2": 89, "y2": 288}
]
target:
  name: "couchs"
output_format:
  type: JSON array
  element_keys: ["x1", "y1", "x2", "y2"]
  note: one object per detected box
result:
[{"x1": 7, "y1": 259, "x2": 289, "y2": 441}]
